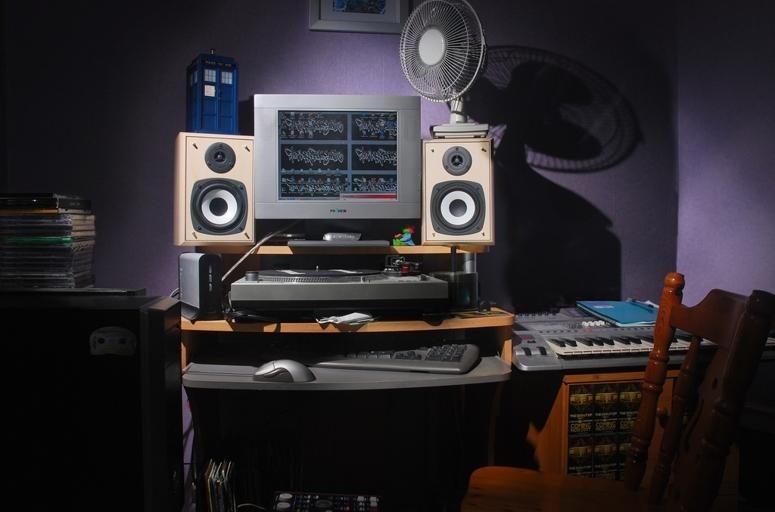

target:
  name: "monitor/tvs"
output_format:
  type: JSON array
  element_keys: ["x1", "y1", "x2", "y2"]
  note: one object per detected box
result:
[{"x1": 254, "y1": 93, "x2": 422, "y2": 222}]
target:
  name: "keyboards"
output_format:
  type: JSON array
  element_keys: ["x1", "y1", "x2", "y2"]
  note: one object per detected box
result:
[{"x1": 306, "y1": 329, "x2": 482, "y2": 375}]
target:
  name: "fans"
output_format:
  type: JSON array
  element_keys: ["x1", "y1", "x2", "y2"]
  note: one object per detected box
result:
[{"x1": 397, "y1": 1, "x2": 492, "y2": 139}]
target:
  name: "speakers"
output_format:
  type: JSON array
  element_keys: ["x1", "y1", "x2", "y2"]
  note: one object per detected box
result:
[
  {"x1": 172, "y1": 131, "x2": 255, "y2": 247},
  {"x1": 421, "y1": 137, "x2": 494, "y2": 246}
]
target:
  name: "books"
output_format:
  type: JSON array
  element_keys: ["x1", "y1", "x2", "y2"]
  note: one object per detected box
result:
[
  {"x1": 575, "y1": 299, "x2": 661, "y2": 329},
  {"x1": 0, "y1": 190, "x2": 99, "y2": 292},
  {"x1": 205, "y1": 457, "x2": 239, "y2": 512}
]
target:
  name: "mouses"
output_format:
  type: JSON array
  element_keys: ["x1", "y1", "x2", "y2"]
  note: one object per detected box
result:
[{"x1": 251, "y1": 357, "x2": 315, "y2": 383}]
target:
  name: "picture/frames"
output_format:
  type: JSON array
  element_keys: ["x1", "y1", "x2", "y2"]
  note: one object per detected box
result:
[{"x1": 305, "y1": 0, "x2": 404, "y2": 37}]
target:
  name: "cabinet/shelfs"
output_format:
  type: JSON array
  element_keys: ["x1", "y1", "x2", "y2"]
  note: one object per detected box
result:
[
  {"x1": 518, "y1": 372, "x2": 674, "y2": 490},
  {"x1": 181, "y1": 246, "x2": 516, "y2": 394}
]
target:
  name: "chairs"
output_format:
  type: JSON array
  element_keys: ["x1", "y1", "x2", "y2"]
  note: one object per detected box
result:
[{"x1": 460, "y1": 273, "x2": 775, "y2": 512}]
